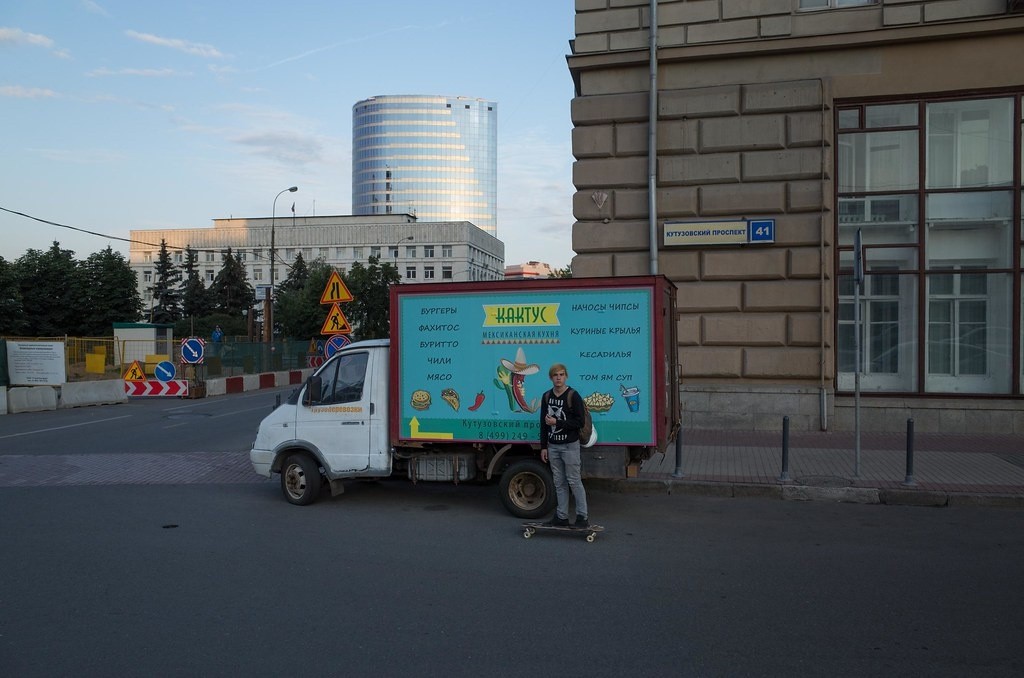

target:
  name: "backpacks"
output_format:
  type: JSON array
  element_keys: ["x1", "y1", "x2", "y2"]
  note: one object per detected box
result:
[{"x1": 546, "y1": 389, "x2": 592, "y2": 445}]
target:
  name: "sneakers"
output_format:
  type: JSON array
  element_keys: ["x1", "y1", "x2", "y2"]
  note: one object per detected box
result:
[
  {"x1": 542, "y1": 513, "x2": 570, "y2": 528},
  {"x1": 575, "y1": 515, "x2": 590, "y2": 528}
]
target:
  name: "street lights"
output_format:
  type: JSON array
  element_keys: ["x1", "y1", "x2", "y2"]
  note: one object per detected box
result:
[{"x1": 269, "y1": 185, "x2": 298, "y2": 343}]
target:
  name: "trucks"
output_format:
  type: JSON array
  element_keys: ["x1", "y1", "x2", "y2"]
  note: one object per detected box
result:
[{"x1": 248, "y1": 274, "x2": 683, "y2": 519}]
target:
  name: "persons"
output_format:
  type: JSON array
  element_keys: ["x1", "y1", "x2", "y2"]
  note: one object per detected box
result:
[
  {"x1": 211, "y1": 325, "x2": 226, "y2": 359},
  {"x1": 540, "y1": 363, "x2": 589, "y2": 528}
]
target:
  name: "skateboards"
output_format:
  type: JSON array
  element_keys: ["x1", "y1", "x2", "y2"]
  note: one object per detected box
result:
[{"x1": 520, "y1": 521, "x2": 606, "y2": 543}]
